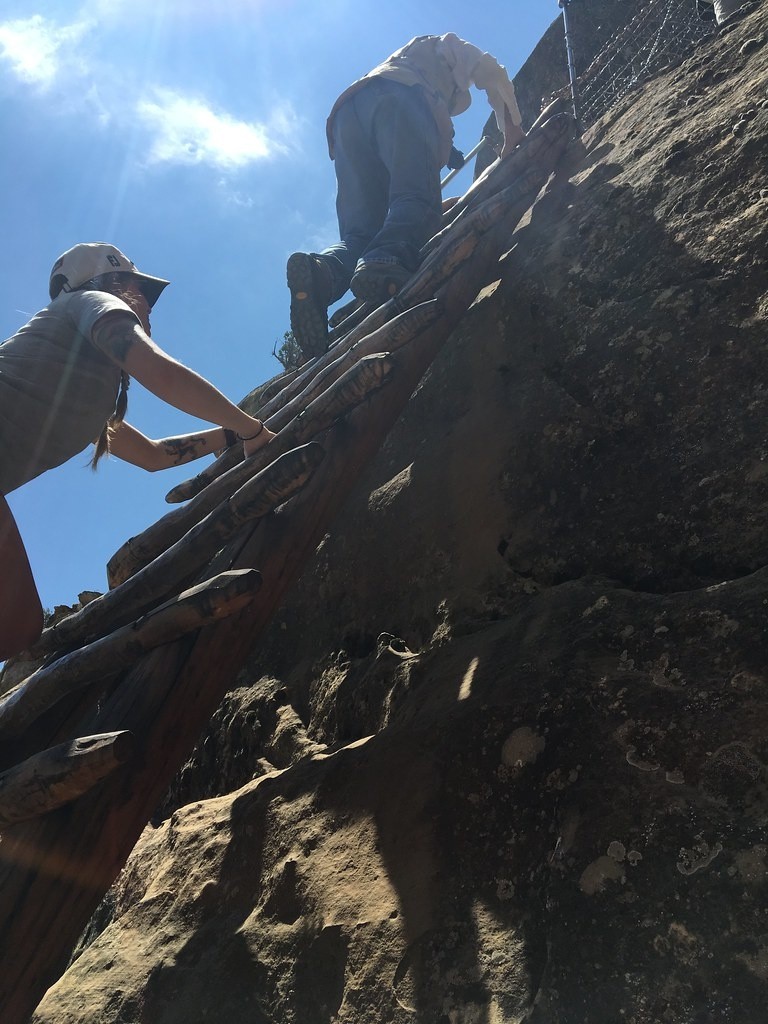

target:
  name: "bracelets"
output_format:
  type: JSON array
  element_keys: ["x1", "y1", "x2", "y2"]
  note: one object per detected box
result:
[
  {"x1": 238, "y1": 419, "x2": 264, "y2": 441},
  {"x1": 223, "y1": 427, "x2": 237, "y2": 447}
]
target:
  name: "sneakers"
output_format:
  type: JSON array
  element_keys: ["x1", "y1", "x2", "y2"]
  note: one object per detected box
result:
[
  {"x1": 350, "y1": 261, "x2": 414, "y2": 304},
  {"x1": 286, "y1": 252, "x2": 333, "y2": 357}
]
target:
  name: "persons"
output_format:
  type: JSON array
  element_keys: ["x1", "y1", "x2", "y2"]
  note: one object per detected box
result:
[
  {"x1": 0, "y1": 243, "x2": 276, "y2": 660},
  {"x1": 287, "y1": 31, "x2": 526, "y2": 358}
]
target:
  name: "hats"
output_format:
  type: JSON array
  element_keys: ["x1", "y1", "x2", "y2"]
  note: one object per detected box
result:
[
  {"x1": 49, "y1": 243, "x2": 171, "y2": 308},
  {"x1": 450, "y1": 89, "x2": 472, "y2": 117}
]
